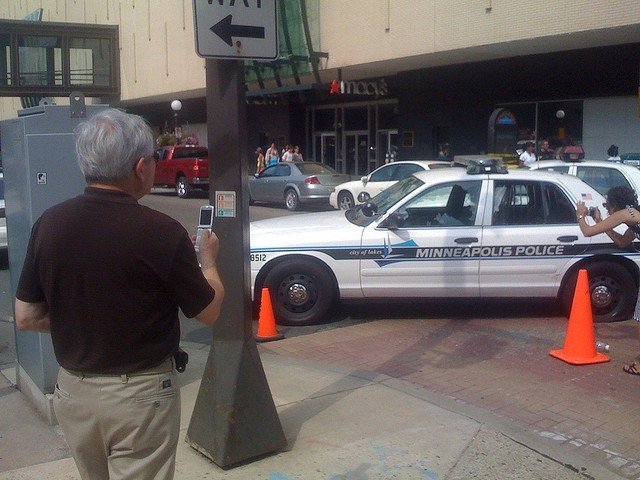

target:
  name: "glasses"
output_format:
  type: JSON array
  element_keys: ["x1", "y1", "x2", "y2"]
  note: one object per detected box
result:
[{"x1": 151, "y1": 151, "x2": 160, "y2": 162}]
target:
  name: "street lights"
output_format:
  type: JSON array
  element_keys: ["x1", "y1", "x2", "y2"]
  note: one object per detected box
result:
[{"x1": 171, "y1": 100, "x2": 182, "y2": 146}]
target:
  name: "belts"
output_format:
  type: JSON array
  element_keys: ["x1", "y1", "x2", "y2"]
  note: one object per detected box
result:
[{"x1": 67, "y1": 356, "x2": 175, "y2": 377}]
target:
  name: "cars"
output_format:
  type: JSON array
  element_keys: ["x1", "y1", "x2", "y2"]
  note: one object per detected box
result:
[
  {"x1": 0, "y1": 173, "x2": 8, "y2": 266},
  {"x1": 248, "y1": 161, "x2": 364, "y2": 210},
  {"x1": 250, "y1": 158, "x2": 640, "y2": 324},
  {"x1": 518, "y1": 146, "x2": 640, "y2": 205},
  {"x1": 329, "y1": 160, "x2": 463, "y2": 211}
]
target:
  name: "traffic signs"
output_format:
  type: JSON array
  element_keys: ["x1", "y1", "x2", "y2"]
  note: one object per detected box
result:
[{"x1": 193, "y1": 0, "x2": 279, "y2": 61}]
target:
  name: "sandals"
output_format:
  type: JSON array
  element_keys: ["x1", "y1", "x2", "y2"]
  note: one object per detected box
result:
[{"x1": 623, "y1": 363, "x2": 640, "y2": 374}]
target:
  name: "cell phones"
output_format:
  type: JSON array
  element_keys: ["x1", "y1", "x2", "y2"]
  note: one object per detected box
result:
[
  {"x1": 587, "y1": 208, "x2": 595, "y2": 217},
  {"x1": 194, "y1": 205, "x2": 214, "y2": 251}
]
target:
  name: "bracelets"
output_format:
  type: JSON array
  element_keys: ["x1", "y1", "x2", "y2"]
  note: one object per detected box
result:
[{"x1": 578, "y1": 214, "x2": 584, "y2": 220}]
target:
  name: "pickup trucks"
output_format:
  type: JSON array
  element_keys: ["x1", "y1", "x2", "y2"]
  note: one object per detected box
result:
[{"x1": 152, "y1": 144, "x2": 208, "y2": 198}]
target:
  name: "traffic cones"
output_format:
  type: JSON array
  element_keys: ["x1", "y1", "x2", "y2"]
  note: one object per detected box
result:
[
  {"x1": 253, "y1": 288, "x2": 286, "y2": 342},
  {"x1": 549, "y1": 269, "x2": 610, "y2": 365}
]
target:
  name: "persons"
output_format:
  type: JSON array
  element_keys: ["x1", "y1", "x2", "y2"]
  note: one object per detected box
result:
[
  {"x1": 265, "y1": 143, "x2": 280, "y2": 167},
  {"x1": 576, "y1": 186, "x2": 640, "y2": 374},
  {"x1": 607, "y1": 145, "x2": 622, "y2": 161},
  {"x1": 15, "y1": 106, "x2": 226, "y2": 480},
  {"x1": 518, "y1": 142, "x2": 536, "y2": 168},
  {"x1": 282, "y1": 144, "x2": 293, "y2": 162},
  {"x1": 281, "y1": 144, "x2": 290, "y2": 157},
  {"x1": 293, "y1": 146, "x2": 304, "y2": 162},
  {"x1": 537, "y1": 140, "x2": 555, "y2": 160},
  {"x1": 256, "y1": 147, "x2": 264, "y2": 174}
]
